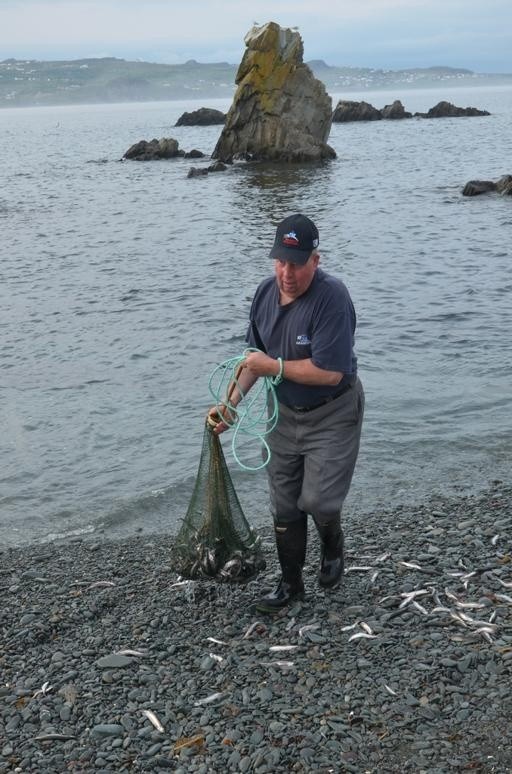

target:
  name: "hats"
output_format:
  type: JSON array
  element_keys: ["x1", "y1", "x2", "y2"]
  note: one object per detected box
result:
[{"x1": 268, "y1": 213, "x2": 319, "y2": 266}]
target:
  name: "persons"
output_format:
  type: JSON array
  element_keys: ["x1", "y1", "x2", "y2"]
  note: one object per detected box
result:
[{"x1": 206, "y1": 211, "x2": 366, "y2": 615}]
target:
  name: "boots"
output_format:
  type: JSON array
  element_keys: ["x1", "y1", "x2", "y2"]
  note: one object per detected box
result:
[
  {"x1": 256, "y1": 521, "x2": 308, "y2": 613},
  {"x1": 312, "y1": 514, "x2": 345, "y2": 589}
]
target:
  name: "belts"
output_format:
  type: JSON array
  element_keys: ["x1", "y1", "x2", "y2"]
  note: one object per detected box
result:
[{"x1": 282, "y1": 377, "x2": 360, "y2": 415}]
